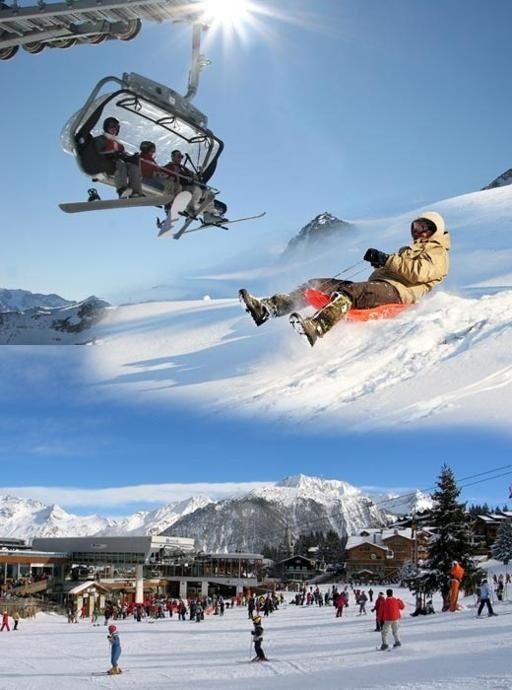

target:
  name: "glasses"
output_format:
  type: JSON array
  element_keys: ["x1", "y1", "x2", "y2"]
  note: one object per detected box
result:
[{"x1": 410, "y1": 220, "x2": 433, "y2": 234}]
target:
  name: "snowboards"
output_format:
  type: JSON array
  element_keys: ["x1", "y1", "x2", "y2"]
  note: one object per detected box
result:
[
  {"x1": 58, "y1": 193, "x2": 174, "y2": 214},
  {"x1": 157, "y1": 191, "x2": 191, "y2": 239}
]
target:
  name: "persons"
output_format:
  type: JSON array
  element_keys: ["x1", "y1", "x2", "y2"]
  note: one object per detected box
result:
[
  {"x1": 251, "y1": 615, "x2": 267, "y2": 661},
  {"x1": 137, "y1": 141, "x2": 181, "y2": 215},
  {"x1": 165, "y1": 149, "x2": 219, "y2": 217},
  {"x1": 238, "y1": 212, "x2": 450, "y2": 348},
  {"x1": 0, "y1": 569, "x2": 511, "y2": 631},
  {"x1": 93, "y1": 117, "x2": 146, "y2": 199},
  {"x1": 106, "y1": 625, "x2": 122, "y2": 676},
  {"x1": 379, "y1": 588, "x2": 404, "y2": 650}
]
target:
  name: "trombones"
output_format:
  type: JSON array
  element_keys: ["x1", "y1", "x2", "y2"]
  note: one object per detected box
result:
[{"x1": 174, "y1": 193, "x2": 267, "y2": 239}]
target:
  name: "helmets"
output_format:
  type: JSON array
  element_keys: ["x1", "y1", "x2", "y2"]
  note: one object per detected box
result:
[
  {"x1": 103, "y1": 117, "x2": 120, "y2": 136},
  {"x1": 252, "y1": 615, "x2": 261, "y2": 623},
  {"x1": 109, "y1": 626, "x2": 116, "y2": 634},
  {"x1": 141, "y1": 141, "x2": 155, "y2": 152}
]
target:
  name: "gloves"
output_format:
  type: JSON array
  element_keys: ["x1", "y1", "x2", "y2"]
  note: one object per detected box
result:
[{"x1": 363, "y1": 248, "x2": 389, "y2": 268}]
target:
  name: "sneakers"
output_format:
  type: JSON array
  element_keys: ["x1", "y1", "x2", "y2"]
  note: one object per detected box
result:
[
  {"x1": 290, "y1": 312, "x2": 318, "y2": 346},
  {"x1": 108, "y1": 666, "x2": 121, "y2": 674},
  {"x1": 381, "y1": 644, "x2": 388, "y2": 650},
  {"x1": 393, "y1": 641, "x2": 401, "y2": 647},
  {"x1": 239, "y1": 288, "x2": 269, "y2": 327},
  {"x1": 117, "y1": 186, "x2": 145, "y2": 199},
  {"x1": 203, "y1": 211, "x2": 224, "y2": 224}
]
